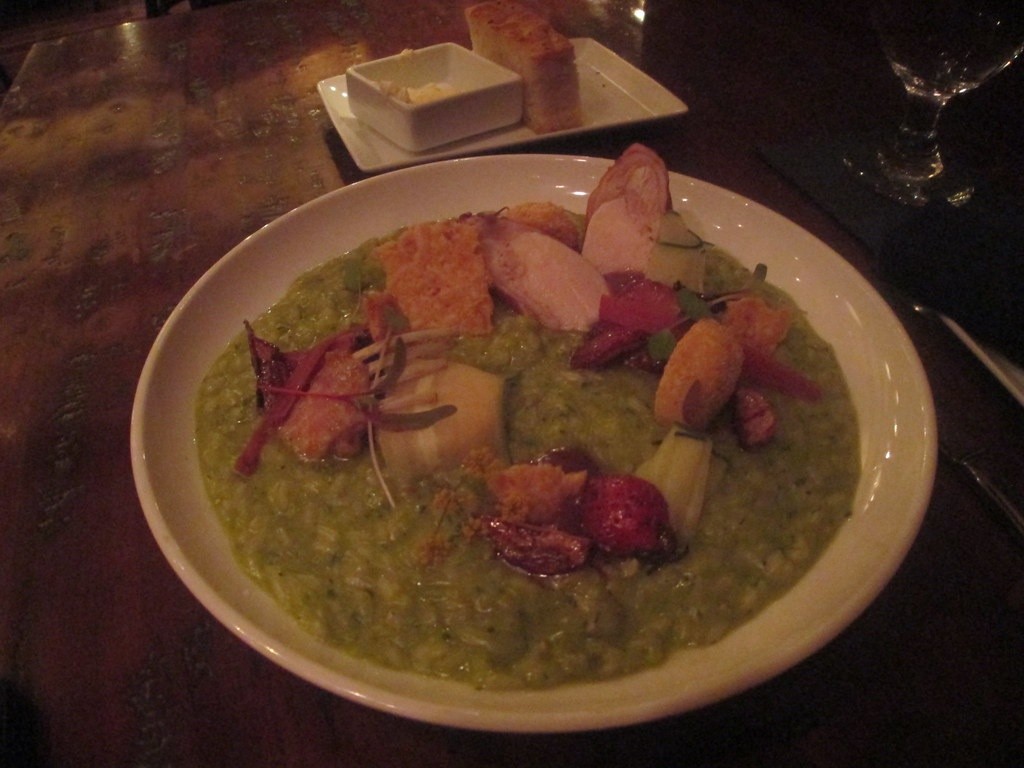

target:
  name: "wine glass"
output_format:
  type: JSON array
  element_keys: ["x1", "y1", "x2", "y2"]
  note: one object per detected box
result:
[{"x1": 839, "y1": 2, "x2": 1024, "y2": 208}]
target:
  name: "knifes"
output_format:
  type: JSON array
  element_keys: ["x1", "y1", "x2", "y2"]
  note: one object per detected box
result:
[{"x1": 713, "y1": 150, "x2": 1022, "y2": 437}]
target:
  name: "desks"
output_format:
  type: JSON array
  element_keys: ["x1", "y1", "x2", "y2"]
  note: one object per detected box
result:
[{"x1": 3, "y1": 2, "x2": 1024, "y2": 768}]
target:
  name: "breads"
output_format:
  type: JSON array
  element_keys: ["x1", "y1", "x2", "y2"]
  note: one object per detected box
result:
[{"x1": 463, "y1": 3, "x2": 582, "y2": 133}]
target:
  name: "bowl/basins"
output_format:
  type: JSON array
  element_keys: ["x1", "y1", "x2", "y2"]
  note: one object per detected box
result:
[{"x1": 347, "y1": 41, "x2": 523, "y2": 155}]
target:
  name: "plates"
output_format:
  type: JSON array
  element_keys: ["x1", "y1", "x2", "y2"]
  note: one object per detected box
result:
[
  {"x1": 131, "y1": 154, "x2": 936, "y2": 734},
  {"x1": 317, "y1": 37, "x2": 689, "y2": 174}
]
